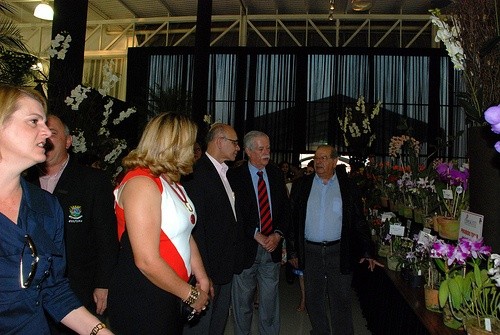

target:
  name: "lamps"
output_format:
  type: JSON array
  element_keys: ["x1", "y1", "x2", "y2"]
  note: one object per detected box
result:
[
  {"x1": 33, "y1": 0, "x2": 54, "y2": 21},
  {"x1": 328, "y1": 0, "x2": 334, "y2": 21},
  {"x1": 352, "y1": 0, "x2": 375, "y2": 11}
]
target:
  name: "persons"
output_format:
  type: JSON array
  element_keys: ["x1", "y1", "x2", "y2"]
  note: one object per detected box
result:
[
  {"x1": 192, "y1": 142, "x2": 202, "y2": 160},
  {"x1": 229, "y1": 131, "x2": 290, "y2": 335},
  {"x1": 180, "y1": 123, "x2": 248, "y2": 335},
  {"x1": 0, "y1": 85, "x2": 115, "y2": 335},
  {"x1": 111, "y1": 110, "x2": 215, "y2": 335},
  {"x1": 254, "y1": 159, "x2": 314, "y2": 313},
  {"x1": 30, "y1": 114, "x2": 117, "y2": 335},
  {"x1": 286, "y1": 144, "x2": 385, "y2": 335}
]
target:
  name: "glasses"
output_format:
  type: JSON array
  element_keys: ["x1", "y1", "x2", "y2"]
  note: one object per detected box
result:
[
  {"x1": 221, "y1": 136, "x2": 239, "y2": 147},
  {"x1": 19, "y1": 234, "x2": 37, "y2": 288}
]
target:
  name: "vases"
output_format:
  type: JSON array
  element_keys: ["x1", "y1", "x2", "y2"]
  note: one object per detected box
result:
[
  {"x1": 414, "y1": 209, "x2": 423, "y2": 222},
  {"x1": 404, "y1": 208, "x2": 413, "y2": 218},
  {"x1": 387, "y1": 252, "x2": 403, "y2": 269},
  {"x1": 410, "y1": 268, "x2": 425, "y2": 288},
  {"x1": 432, "y1": 216, "x2": 439, "y2": 231},
  {"x1": 398, "y1": 204, "x2": 404, "y2": 215},
  {"x1": 438, "y1": 217, "x2": 460, "y2": 240},
  {"x1": 424, "y1": 287, "x2": 443, "y2": 313},
  {"x1": 443, "y1": 301, "x2": 470, "y2": 330},
  {"x1": 379, "y1": 244, "x2": 389, "y2": 257},
  {"x1": 466, "y1": 315, "x2": 500, "y2": 335},
  {"x1": 381, "y1": 196, "x2": 390, "y2": 207}
]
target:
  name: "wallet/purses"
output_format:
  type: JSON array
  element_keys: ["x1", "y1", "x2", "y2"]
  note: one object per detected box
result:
[{"x1": 178, "y1": 275, "x2": 197, "y2": 314}]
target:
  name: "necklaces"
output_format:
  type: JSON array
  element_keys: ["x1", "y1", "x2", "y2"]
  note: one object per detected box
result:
[{"x1": 160, "y1": 174, "x2": 194, "y2": 212}]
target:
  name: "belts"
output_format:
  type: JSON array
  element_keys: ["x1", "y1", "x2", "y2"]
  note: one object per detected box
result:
[{"x1": 305, "y1": 240, "x2": 341, "y2": 247}]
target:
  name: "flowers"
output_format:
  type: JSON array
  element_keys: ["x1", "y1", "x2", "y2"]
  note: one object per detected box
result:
[
  {"x1": 428, "y1": 9, "x2": 485, "y2": 126},
  {"x1": 338, "y1": 96, "x2": 500, "y2": 335},
  {"x1": 203, "y1": 114, "x2": 221, "y2": 129},
  {"x1": 63, "y1": 58, "x2": 137, "y2": 185},
  {"x1": 31, "y1": 31, "x2": 71, "y2": 78}
]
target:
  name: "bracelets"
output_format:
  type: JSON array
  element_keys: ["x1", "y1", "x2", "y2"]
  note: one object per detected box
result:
[
  {"x1": 90, "y1": 322, "x2": 107, "y2": 335},
  {"x1": 183, "y1": 286, "x2": 200, "y2": 305}
]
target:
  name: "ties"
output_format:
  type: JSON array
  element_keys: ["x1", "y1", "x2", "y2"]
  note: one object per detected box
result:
[{"x1": 257, "y1": 171, "x2": 272, "y2": 237}]
